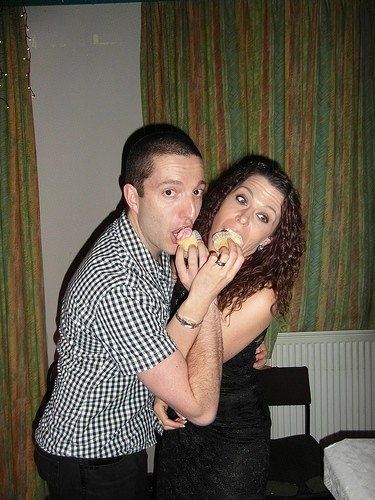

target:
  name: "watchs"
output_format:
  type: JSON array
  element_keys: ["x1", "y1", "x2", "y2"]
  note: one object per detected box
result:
[{"x1": 176, "y1": 312, "x2": 203, "y2": 330}]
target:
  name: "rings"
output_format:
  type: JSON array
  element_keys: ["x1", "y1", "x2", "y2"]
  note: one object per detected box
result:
[{"x1": 217, "y1": 260, "x2": 225, "y2": 266}]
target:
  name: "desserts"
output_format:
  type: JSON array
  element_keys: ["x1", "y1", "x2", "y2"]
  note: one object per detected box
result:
[
  {"x1": 213, "y1": 230, "x2": 244, "y2": 251},
  {"x1": 176, "y1": 230, "x2": 202, "y2": 259}
]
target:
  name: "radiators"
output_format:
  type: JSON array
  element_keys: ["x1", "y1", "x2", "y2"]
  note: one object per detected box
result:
[{"x1": 267, "y1": 330, "x2": 375, "y2": 441}]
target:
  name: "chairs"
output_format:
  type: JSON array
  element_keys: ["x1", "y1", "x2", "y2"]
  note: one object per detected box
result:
[{"x1": 255, "y1": 366, "x2": 325, "y2": 500}]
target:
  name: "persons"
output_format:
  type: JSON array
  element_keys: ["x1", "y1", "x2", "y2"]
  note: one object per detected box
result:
[
  {"x1": 32, "y1": 123, "x2": 223, "y2": 500},
  {"x1": 154, "y1": 155, "x2": 307, "y2": 500}
]
